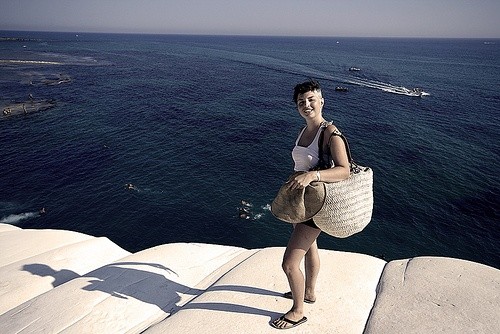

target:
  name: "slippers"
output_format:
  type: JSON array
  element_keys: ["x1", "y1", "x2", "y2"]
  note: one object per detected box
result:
[
  {"x1": 282, "y1": 290, "x2": 316, "y2": 304},
  {"x1": 270, "y1": 315, "x2": 307, "y2": 330}
]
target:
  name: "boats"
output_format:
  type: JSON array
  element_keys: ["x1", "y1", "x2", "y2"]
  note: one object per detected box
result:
[
  {"x1": 349, "y1": 67, "x2": 360, "y2": 71},
  {"x1": 405, "y1": 89, "x2": 430, "y2": 96},
  {"x1": 335, "y1": 88, "x2": 349, "y2": 93}
]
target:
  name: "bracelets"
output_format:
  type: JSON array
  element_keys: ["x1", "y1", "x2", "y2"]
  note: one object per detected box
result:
[{"x1": 316, "y1": 171, "x2": 321, "y2": 181}]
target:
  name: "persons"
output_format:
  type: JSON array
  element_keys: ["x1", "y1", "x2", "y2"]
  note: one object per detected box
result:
[{"x1": 272, "y1": 80, "x2": 351, "y2": 329}]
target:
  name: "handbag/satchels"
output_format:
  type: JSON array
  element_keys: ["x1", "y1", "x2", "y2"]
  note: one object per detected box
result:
[{"x1": 310, "y1": 131, "x2": 373, "y2": 238}]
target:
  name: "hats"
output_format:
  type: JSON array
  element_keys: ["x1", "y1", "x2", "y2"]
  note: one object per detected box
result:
[{"x1": 271, "y1": 171, "x2": 326, "y2": 223}]
